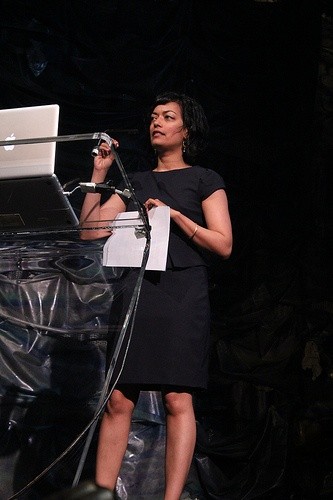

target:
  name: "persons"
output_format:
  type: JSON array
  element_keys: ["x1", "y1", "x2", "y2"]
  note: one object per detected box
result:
[{"x1": 81, "y1": 92, "x2": 233, "y2": 500}]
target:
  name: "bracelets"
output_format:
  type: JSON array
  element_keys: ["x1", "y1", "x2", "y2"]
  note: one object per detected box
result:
[{"x1": 190, "y1": 223, "x2": 197, "y2": 241}]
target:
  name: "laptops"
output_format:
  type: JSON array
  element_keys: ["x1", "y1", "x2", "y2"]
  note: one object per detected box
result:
[{"x1": 0, "y1": 105, "x2": 79, "y2": 227}]
